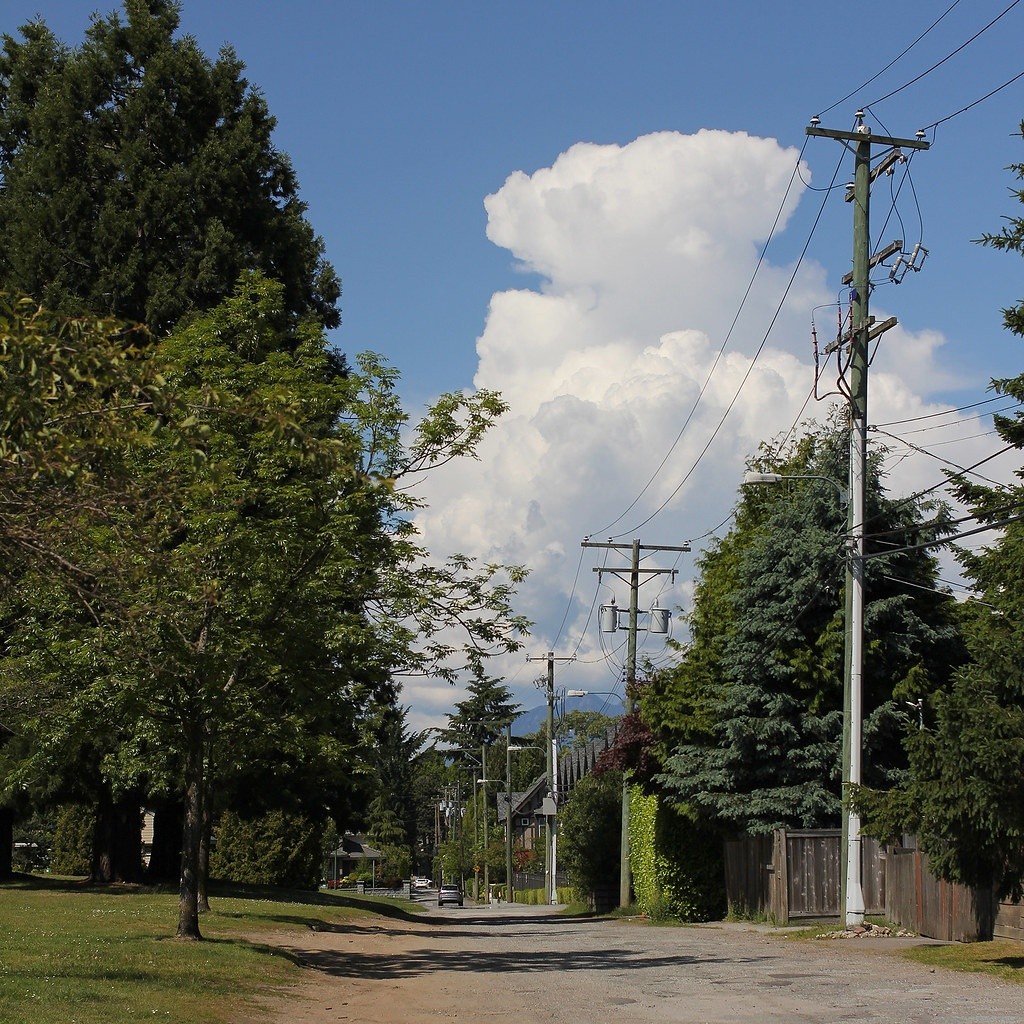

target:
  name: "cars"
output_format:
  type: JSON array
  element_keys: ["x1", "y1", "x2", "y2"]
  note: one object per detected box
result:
[{"x1": 415, "y1": 879, "x2": 431, "y2": 889}]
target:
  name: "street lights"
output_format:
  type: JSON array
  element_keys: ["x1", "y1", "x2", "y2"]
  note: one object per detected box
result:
[
  {"x1": 476, "y1": 778, "x2": 513, "y2": 903},
  {"x1": 508, "y1": 745, "x2": 550, "y2": 905},
  {"x1": 567, "y1": 689, "x2": 632, "y2": 909},
  {"x1": 743, "y1": 469, "x2": 866, "y2": 933}
]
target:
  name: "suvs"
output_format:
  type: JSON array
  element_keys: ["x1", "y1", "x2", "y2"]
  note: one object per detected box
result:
[{"x1": 437, "y1": 883, "x2": 464, "y2": 907}]
